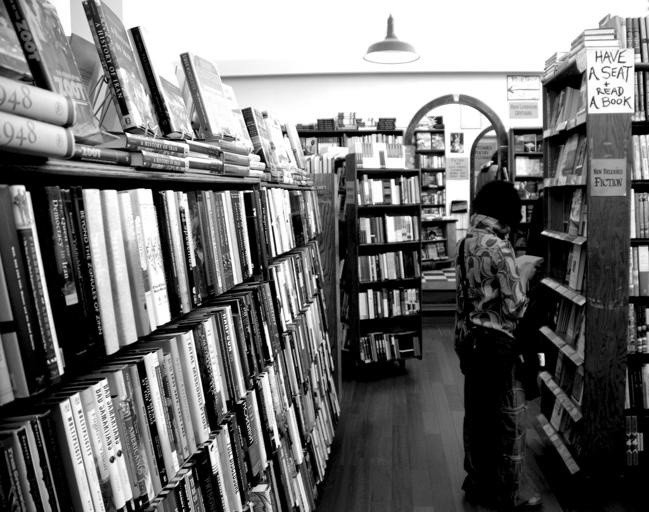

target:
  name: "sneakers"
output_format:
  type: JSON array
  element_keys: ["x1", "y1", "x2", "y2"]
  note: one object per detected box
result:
[{"x1": 516, "y1": 493, "x2": 543, "y2": 509}]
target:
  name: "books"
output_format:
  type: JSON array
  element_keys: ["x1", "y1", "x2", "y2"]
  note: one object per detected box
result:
[
  {"x1": 306, "y1": 113, "x2": 443, "y2": 369},
  {"x1": 1, "y1": 2, "x2": 340, "y2": 511},
  {"x1": 510, "y1": 14, "x2": 648, "y2": 488}
]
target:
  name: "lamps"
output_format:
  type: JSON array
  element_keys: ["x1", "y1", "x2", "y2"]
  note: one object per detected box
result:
[{"x1": 363, "y1": 13, "x2": 420, "y2": 66}]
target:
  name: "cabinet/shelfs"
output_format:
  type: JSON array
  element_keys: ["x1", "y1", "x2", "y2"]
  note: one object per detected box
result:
[{"x1": 413, "y1": 114, "x2": 455, "y2": 268}]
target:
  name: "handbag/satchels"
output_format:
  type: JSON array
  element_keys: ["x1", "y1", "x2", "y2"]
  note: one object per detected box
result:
[{"x1": 459, "y1": 321, "x2": 518, "y2": 382}]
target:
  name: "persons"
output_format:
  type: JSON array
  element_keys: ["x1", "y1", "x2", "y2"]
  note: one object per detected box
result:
[{"x1": 453, "y1": 181, "x2": 543, "y2": 511}]
